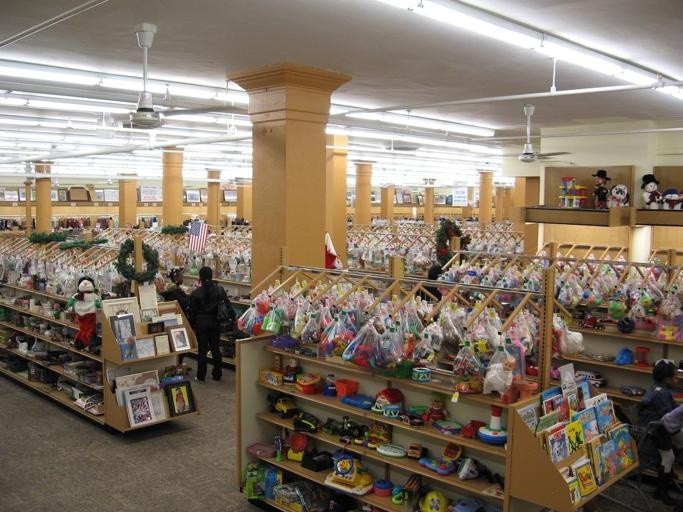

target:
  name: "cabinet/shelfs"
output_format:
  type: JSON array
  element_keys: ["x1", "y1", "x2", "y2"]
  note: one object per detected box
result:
[{"x1": 0, "y1": 228, "x2": 683, "y2": 511}]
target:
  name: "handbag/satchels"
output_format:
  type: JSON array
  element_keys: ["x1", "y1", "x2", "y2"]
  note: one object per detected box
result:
[{"x1": 215, "y1": 300, "x2": 230, "y2": 321}]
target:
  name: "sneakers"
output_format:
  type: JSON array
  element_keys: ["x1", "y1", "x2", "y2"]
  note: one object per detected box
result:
[
  {"x1": 212, "y1": 376, "x2": 222, "y2": 381},
  {"x1": 194, "y1": 376, "x2": 205, "y2": 384}
]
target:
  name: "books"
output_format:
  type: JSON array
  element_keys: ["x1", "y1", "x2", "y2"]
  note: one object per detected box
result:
[{"x1": 517, "y1": 363, "x2": 633, "y2": 504}]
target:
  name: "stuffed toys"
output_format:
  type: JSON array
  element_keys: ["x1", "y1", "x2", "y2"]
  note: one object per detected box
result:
[
  {"x1": 68, "y1": 276, "x2": 101, "y2": 354},
  {"x1": 607, "y1": 183, "x2": 628, "y2": 208},
  {"x1": 661, "y1": 189, "x2": 683, "y2": 210},
  {"x1": 641, "y1": 173, "x2": 662, "y2": 209}
]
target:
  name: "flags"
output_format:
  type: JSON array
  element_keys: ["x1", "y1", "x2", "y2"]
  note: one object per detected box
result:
[{"x1": 188, "y1": 220, "x2": 207, "y2": 255}]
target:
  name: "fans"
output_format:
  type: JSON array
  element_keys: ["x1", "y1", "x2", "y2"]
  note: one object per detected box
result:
[
  {"x1": 112, "y1": 22, "x2": 244, "y2": 129},
  {"x1": 496, "y1": 103, "x2": 574, "y2": 164}
]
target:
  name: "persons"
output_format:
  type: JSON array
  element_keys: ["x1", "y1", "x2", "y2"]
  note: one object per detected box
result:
[
  {"x1": 159, "y1": 268, "x2": 193, "y2": 372},
  {"x1": 645, "y1": 404, "x2": 682, "y2": 511},
  {"x1": 415, "y1": 265, "x2": 443, "y2": 301},
  {"x1": 189, "y1": 266, "x2": 236, "y2": 384},
  {"x1": 426, "y1": 394, "x2": 448, "y2": 422},
  {"x1": 636, "y1": 358, "x2": 680, "y2": 506},
  {"x1": 591, "y1": 169, "x2": 607, "y2": 209}
]
